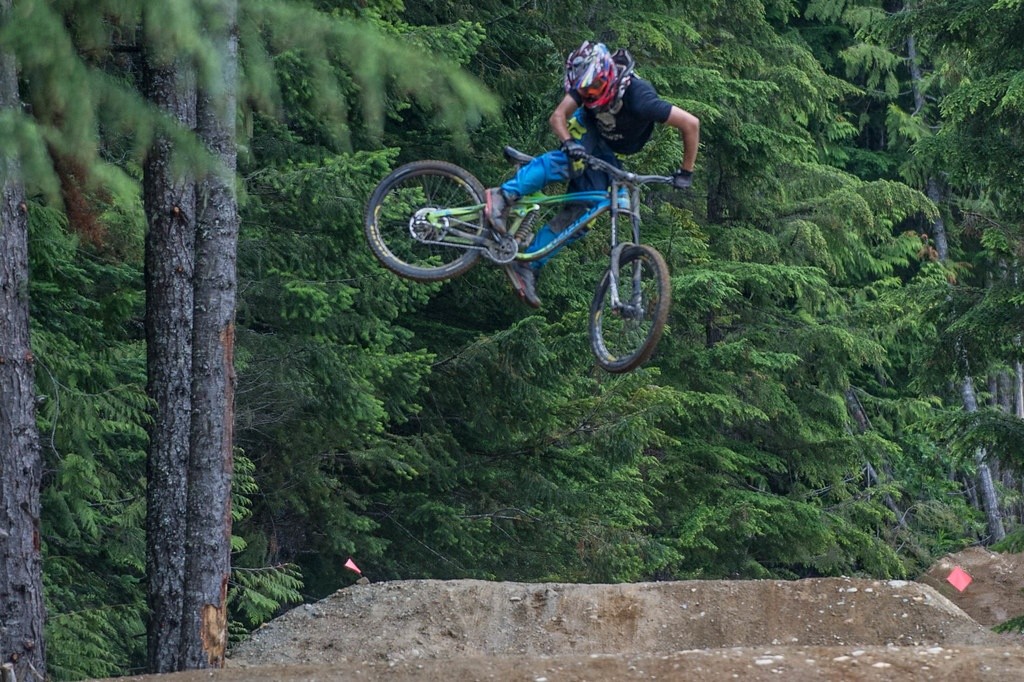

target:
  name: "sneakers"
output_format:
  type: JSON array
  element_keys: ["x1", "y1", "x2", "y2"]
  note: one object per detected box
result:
[
  {"x1": 485, "y1": 186, "x2": 512, "y2": 234},
  {"x1": 506, "y1": 263, "x2": 541, "y2": 310}
]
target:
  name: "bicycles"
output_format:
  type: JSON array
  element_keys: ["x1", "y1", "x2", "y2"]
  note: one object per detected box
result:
[{"x1": 363, "y1": 145, "x2": 680, "y2": 375}]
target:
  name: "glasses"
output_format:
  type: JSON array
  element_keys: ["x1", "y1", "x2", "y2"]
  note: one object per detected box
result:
[{"x1": 576, "y1": 76, "x2": 607, "y2": 99}]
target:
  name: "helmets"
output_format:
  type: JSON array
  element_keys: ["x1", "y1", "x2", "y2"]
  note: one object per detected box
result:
[{"x1": 564, "y1": 40, "x2": 617, "y2": 109}]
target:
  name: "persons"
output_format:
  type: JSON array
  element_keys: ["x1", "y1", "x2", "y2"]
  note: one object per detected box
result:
[{"x1": 483, "y1": 39, "x2": 699, "y2": 308}]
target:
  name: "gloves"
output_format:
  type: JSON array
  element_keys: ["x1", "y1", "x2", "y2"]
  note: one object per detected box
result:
[
  {"x1": 561, "y1": 138, "x2": 585, "y2": 161},
  {"x1": 671, "y1": 168, "x2": 693, "y2": 190}
]
